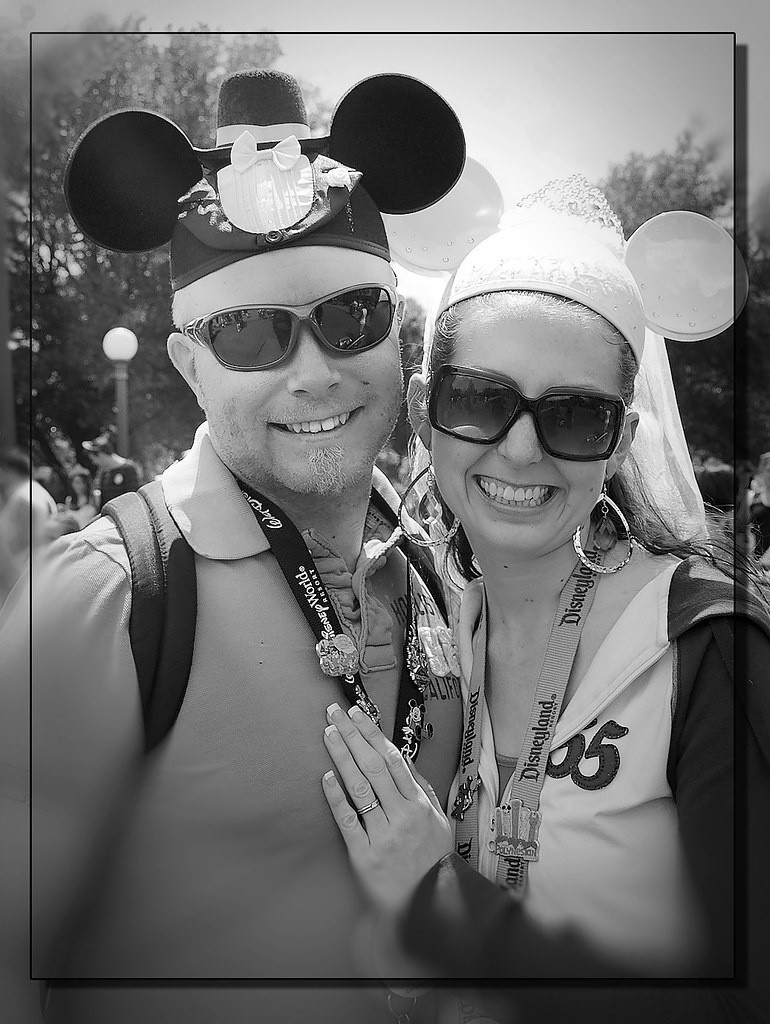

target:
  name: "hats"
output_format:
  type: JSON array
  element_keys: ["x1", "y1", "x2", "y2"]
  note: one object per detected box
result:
[
  {"x1": 375, "y1": 153, "x2": 746, "y2": 370},
  {"x1": 61, "y1": 69, "x2": 466, "y2": 295}
]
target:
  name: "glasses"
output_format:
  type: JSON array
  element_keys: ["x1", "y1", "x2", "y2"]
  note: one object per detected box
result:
[
  {"x1": 178, "y1": 282, "x2": 404, "y2": 374},
  {"x1": 426, "y1": 364, "x2": 626, "y2": 463}
]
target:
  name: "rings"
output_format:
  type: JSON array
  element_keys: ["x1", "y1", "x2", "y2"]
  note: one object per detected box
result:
[{"x1": 357, "y1": 798, "x2": 380, "y2": 817}]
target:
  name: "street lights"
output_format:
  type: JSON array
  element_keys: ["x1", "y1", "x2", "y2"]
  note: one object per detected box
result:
[{"x1": 103, "y1": 324, "x2": 139, "y2": 457}]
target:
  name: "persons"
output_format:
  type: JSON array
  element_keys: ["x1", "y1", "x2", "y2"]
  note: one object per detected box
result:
[
  {"x1": 209, "y1": 308, "x2": 249, "y2": 343},
  {"x1": 314, "y1": 297, "x2": 376, "y2": 336},
  {"x1": 601, "y1": 407, "x2": 611, "y2": 433},
  {"x1": 0, "y1": 435, "x2": 139, "y2": 605},
  {"x1": 0, "y1": 151, "x2": 465, "y2": 1024},
  {"x1": 447, "y1": 395, "x2": 505, "y2": 414},
  {"x1": 320, "y1": 218, "x2": 768, "y2": 1024}
]
